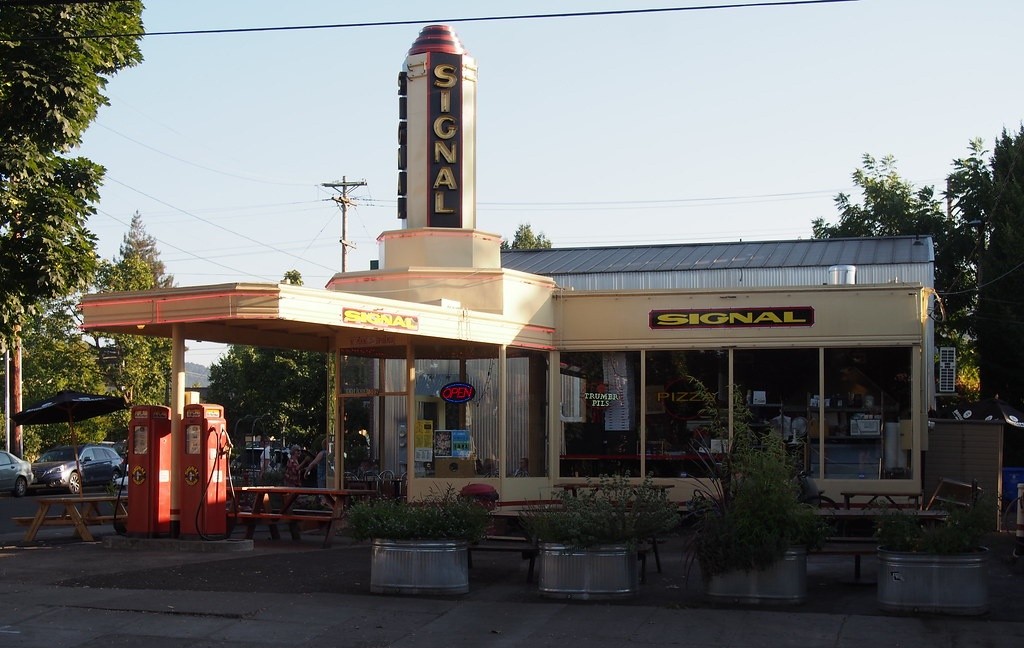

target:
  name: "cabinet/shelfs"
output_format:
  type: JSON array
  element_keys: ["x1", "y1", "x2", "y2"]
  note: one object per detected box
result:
[
  {"x1": 804, "y1": 391, "x2": 885, "y2": 478},
  {"x1": 734, "y1": 397, "x2": 784, "y2": 451}
]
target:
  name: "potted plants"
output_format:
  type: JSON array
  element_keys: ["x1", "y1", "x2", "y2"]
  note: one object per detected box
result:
[
  {"x1": 873, "y1": 477, "x2": 1011, "y2": 617},
  {"x1": 518, "y1": 476, "x2": 683, "y2": 604},
  {"x1": 334, "y1": 484, "x2": 504, "y2": 597},
  {"x1": 673, "y1": 374, "x2": 839, "y2": 606}
]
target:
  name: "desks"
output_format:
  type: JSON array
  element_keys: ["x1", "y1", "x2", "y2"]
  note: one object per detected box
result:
[
  {"x1": 806, "y1": 509, "x2": 951, "y2": 537},
  {"x1": 23, "y1": 496, "x2": 129, "y2": 542},
  {"x1": 560, "y1": 453, "x2": 726, "y2": 478},
  {"x1": 553, "y1": 482, "x2": 675, "y2": 512},
  {"x1": 467, "y1": 511, "x2": 662, "y2": 584},
  {"x1": 839, "y1": 491, "x2": 923, "y2": 510},
  {"x1": 225, "y1": 483, "x2": 378, "y2": 549}
]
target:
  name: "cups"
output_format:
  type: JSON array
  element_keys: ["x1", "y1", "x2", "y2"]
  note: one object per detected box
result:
[{"x1": 853, "y1": 393, "x2": 862, "y2": 408}]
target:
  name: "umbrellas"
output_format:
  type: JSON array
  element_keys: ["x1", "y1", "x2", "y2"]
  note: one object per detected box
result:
[{"x1": 10, "y1": 389, "x2": 127, "y2": 516}]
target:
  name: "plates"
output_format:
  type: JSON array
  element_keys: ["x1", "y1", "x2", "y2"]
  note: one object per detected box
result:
[{"x1": 791, "y1": 417, "x2": 808, "y2": 436}]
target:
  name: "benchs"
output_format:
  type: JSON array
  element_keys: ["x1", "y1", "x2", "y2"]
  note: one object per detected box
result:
[
  {"x1": 827, "y1": 537, "x2": 886, "y2": 543},
  {"x1": 227, "y1": 508, "x2": 350, "y2": 521},
  {"x1": 808, "y1": 549, "x2": 862, "y2": 579},
  {"x1": 482, "y1": 534, "x2": 670, "y2": 545},
  {"x1": 467, "y1": 544, "x2": 653, "y2": 552},
  {"x1": 86, "y1": 515, "x2": 129, "y2": 521},
  {"x1": 11, "y1": 515, "x2": 72, "y2": 521}
]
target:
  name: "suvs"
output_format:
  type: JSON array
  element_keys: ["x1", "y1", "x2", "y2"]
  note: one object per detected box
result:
[
  {"x1": 29, "y1": 444, "x2": 126, "y2": 495},
  {"x1": 231, "y1": 446, "x2": 318, "y2": 488}
]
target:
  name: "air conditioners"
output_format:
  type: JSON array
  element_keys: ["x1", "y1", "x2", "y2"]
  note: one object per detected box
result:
[{"x1": 939, "y1": 346, "x2": 956, "y2": 392}]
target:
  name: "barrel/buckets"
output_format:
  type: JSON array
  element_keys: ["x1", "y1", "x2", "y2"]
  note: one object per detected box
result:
[{"x1": 1002, "y1": 467, "x2": 1024, "y2": 512}]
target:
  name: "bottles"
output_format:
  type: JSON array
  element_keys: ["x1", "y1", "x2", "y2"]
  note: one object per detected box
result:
[{"x1": 746, "y1": 389, "x2": 752, "y2": 405}]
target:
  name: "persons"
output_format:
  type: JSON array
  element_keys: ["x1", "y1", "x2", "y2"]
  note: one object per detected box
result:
[
  {"x1": 259, "y1": 436, "x2": 329, "y2": 515},
  {"x1": 514, "y1": 458, "x2": 528, "y2": 477}
]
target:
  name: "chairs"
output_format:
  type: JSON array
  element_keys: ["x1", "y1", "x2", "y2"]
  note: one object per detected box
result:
[{"x1": 344, "y1": 470, "x2": 408, "y2": 497}]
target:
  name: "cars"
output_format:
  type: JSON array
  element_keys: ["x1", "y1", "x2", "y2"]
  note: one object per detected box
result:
[
  {"x1": 111, "y1": 442, "x2": 129, "y2": 465},
  {"x1": 0, "y1": 450, "x2": 34, "y2": 499}
]
